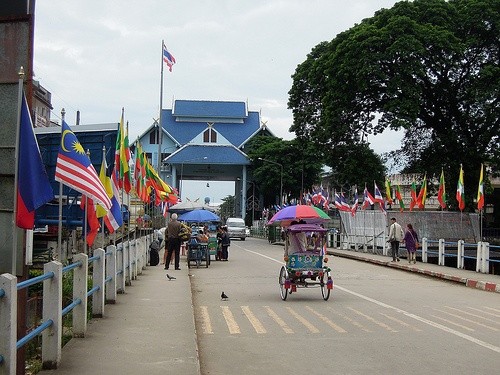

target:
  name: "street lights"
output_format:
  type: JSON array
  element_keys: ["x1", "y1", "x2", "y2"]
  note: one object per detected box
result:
[
  {"x1": 237, "y1": 177, "x2": 255, "y2": 222},
  {"x1": 259, "y1": 158, "x2": 283, "y2": 209}
]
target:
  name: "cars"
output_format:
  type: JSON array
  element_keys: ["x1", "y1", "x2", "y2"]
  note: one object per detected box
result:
[{"x1": 245, "y1": 229, "x2": 251, "y2": 237}]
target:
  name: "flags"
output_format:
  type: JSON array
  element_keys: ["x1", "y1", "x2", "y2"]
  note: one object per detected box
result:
[
  {"x1": 14, "y1": 93, "x2": 178, "y2": 246},
  {"x1": 163, "y1": 44, "x2": 175, "y2": 71},
  {"x1": 264, "y1": 165, "x2": 484, "y2": 212}
]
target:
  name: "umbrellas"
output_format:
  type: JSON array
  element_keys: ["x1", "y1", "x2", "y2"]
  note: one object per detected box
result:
[
  {"x1": 176, "y1": 209, "x2": 221, "y2": 234},
  {"x1": 268, "y1": 204, "x2": 330, "y2": 226}
]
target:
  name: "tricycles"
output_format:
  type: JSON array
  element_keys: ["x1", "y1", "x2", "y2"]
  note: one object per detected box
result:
[
  {"x1": 187, "y1": 236, "x2": 209, "y2": 269},
  {"x1": 278, "y1": 224, "x2": 331, "y2": 301}
]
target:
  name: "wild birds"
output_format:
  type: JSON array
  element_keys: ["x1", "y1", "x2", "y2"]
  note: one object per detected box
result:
[
  {"x1": 188, "y1": 273, "x2": 194, "y2": 277},
  {"x1": 167, "y1": 274, "x2": 177, "y2": 280},
  {"x1": 220, "y1": 291, "x2": 230, "y2": 301}
]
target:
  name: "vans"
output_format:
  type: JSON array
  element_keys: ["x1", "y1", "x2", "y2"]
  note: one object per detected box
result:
[{"x1": 226, "y1": 218, "x2": 247, "y2": 241}]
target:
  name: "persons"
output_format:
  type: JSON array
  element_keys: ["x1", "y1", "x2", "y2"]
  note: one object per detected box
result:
[
  {"x1": 135, "y1": 214, "x2": 152, "y2": 228},
  {"x1": 199, "y1": 225, "x2": 230, "y2": 261},
  {"x1": 387, "y1": 217, "x2": 405, "y2": 262},
  {"x1": 164, "y1": 214, "x2": 182, "y2": 270},
  {"x1": 287, "y1": 219, "x2": 307, "y2": 257},
  {"x1": 403, "y1": 224, "x2": 419, "y2": 264}
]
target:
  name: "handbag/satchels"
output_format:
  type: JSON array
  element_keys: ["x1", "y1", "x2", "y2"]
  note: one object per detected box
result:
[
  {"x1": 415, "y1": 241, "x2": 418, "y2": 248},
  {"x1": 390, "y1": 237, "x2": 396, "y2": 243}
]
